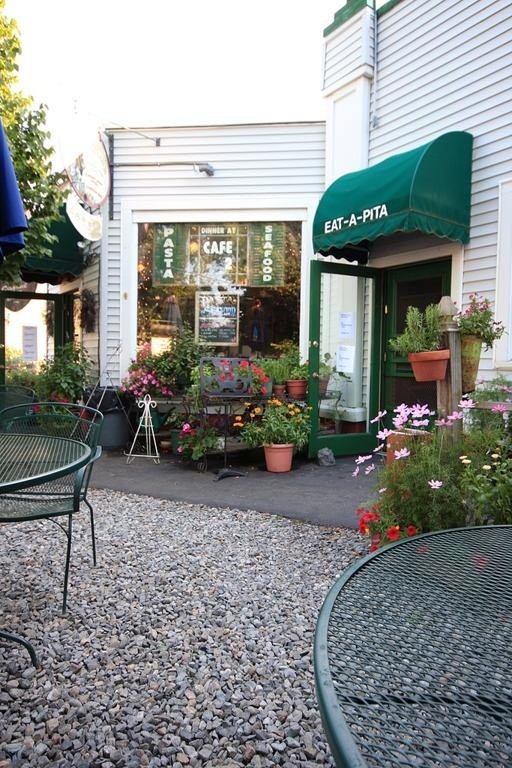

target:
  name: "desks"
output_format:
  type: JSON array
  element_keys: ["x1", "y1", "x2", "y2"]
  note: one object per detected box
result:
[
  {"x1": 312, "y1": 522, "x2": 511, "y2": 768},
  {"x1": 1, "y1": 433, "x2": 92, "y2": 669}
]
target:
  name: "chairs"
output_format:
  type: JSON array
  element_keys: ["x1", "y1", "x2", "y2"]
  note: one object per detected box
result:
[
  {"x1": 0, "y1": 401, "x2": 105, "y2": 614},
  {"x1": 0, "y1": 384, "x2": 35, "y2": 417}
]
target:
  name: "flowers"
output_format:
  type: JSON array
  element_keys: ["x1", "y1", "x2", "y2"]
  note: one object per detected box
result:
[
  {"x1": 457, "y1": 292, "x2": 510, "y2": 351},
  {"x1": 351, "y1": 377, "x2": 512, "y2": 567},
  {"x1": 28, "y1": 393, "x2": 89, "y2": 441},
  {"x1": 119, "y1": 368, "x2": 174, "y2": 399},
  {"x1": 177, "y1": 422, "x2": 219, "y2": 460},
  {"x1": 219, "y1": 357, "x2": 270, "y2": 396},
  {"x1": 232, "y1": 399, "x2": 313, "y2": 453}
]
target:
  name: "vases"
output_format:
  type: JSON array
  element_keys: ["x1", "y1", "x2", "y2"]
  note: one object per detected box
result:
[
  {"x1": 262, "y1": 443, "x2": 294, "y2": 473},
  {"x1": 197, "y1": 456, "x2": 205, "y2": 471},
  {"x1": 460, "y1": 334, "x2": 482, "y2": 394}
]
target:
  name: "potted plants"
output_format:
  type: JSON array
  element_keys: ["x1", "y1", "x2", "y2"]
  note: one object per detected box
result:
[
  {"x1": 257, "y1": 338, "x2": 335, "y2": 401},
  {"x1": 389, "y1": 302, "x2": 450, "y2": 382},
  {"x1": 164, "y1": 412, "x2": 189, "y2": 456}
]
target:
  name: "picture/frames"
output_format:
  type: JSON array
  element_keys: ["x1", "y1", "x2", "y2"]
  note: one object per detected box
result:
[{"x1": 194, "y1": 291, "x2": 240, "y2": 346}]
